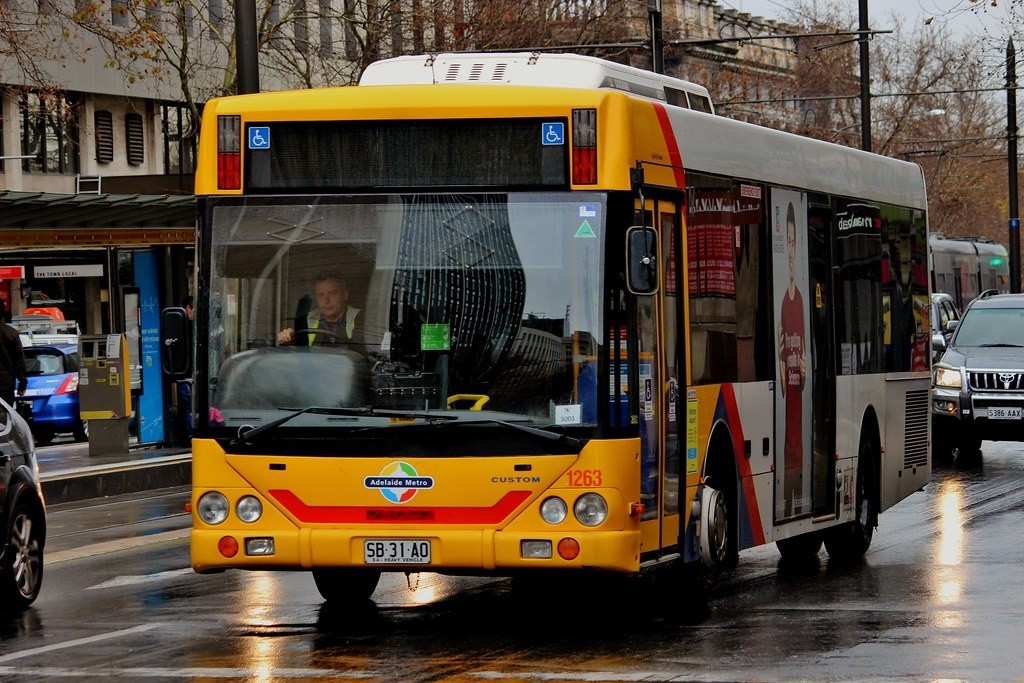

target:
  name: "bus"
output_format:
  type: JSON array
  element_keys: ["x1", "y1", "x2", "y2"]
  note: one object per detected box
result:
[
  {"x1": 928, "y1": 231, "x2": 1011, "y2": 314},
  {"x1": 185, "y1": 50, "x2": 938, "y2": 610}
]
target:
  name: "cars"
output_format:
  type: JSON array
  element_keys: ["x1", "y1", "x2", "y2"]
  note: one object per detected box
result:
[
  {"x1": 928, "y1": 292, "x2": 960, "y2": 364},
  {"x1": 0, "y1": 393, "x2": 50, "y2": 618},
  {"x1": 18, "y1": 343, "x2": 89, "y2": 442}
]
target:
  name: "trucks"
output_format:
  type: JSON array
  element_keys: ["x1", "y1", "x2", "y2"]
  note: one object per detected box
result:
[{"x1": 3, "y1": 313, "x2": 80, "y2": 346}]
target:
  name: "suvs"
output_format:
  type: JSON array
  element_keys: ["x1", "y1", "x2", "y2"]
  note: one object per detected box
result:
[{"x1": 932, "y1": 287, "x2": 1024, "y2": 468}]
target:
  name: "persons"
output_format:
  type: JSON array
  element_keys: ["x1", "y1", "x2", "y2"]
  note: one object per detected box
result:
[
  {"x1": 278, "y1": 269, "x2": 367, "y2": 349},
  {"x1": 0, "y1": 298, "x2": 28, "y2": 409},
  {"x1": 160, "y1": 296, "x2": 193, "y2": 449}
]
